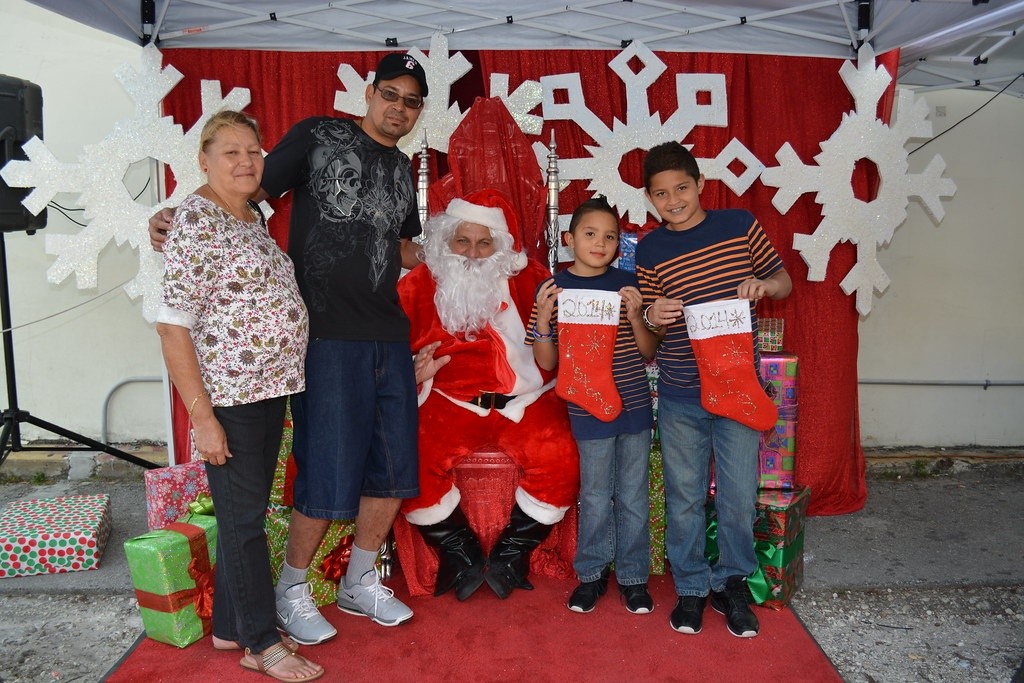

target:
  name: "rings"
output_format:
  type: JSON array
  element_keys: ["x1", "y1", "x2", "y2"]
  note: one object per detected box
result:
[{"x1": 200, "y1": 457, "x2": 208, "y2": 461}]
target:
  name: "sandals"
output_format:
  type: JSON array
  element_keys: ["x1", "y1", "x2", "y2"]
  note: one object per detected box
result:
[
  {"x1": 240, "y1": 644, "x2": 325, "y2": 683},
  {"x1": 212, "y1": 633, "x2": 299, "y2": 653}
]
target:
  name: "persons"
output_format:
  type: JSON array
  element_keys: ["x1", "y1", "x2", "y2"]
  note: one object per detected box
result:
[
  {"x1": 634, "y1": 141, "x2": 792, "y2": 637},
  {"x1": 148, "y1": 53, "x2": 429, "y2": 646},
  {"x1": 156, "y1": 110, "x2": 324, "y2": 683},
  {"x1": 525, "y1": 198, "x2": 660, "y2": 612},
  {"x1": 396, "y1": 189, "x2": 580, "y2": 601}
]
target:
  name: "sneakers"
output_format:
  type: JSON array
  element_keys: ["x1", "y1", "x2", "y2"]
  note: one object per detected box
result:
[
  {"x1": 567, "y1": 567, "x2": 610, "y2": 613},
  {"x1": 336, "y1": 564, "x2": 414, "y2": 627},
  {"x1": 670, "y1": 596, "x2": 708, "y2": 634},
  {"x1": 617, "y1": 583, "x2": 655, "y2": 614},
  {"x1": 712, "y1": 575, "x2": 760, "y2": 638},
  {"x1": 275, "y1": 580, "x2": 338, "y2": 646}
]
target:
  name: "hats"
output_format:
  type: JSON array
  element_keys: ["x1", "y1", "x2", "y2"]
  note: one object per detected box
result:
[
  {"x1": 374, "y1": 53, "x2": 429, "y2": 97},
  {"x1": 445, "y1": 189, "x2": 528, "y2": 271}
]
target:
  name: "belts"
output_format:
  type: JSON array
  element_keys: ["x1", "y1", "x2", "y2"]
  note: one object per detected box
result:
[{"x1": 468, "y1": 392, "x2": 518, "y2": 410}]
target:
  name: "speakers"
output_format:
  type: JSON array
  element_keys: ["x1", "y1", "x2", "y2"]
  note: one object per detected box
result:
[{"x1": 0, "y1": 74, "x2": 47, "y2": 234}]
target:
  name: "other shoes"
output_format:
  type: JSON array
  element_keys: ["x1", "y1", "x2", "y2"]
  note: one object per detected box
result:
[
  {"x1": 484, "y1": 559, "x2": 517, "y2": 600},
  {"x1": 454, "y1": 557, "x2": 487, "y2": 602}
]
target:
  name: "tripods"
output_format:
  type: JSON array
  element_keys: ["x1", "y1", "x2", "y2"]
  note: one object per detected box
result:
[{"x1": 0, "y1": 232, "x2": 164, "y2": 470}]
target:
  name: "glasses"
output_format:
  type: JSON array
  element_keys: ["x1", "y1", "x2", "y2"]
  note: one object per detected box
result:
[{"x1": 372, "y1": 84, "x2": 423, "y2": 110}]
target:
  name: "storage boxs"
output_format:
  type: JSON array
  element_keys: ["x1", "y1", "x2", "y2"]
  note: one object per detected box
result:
[
  {"x1": 0, "y1": 493, "x2": 112, "y2": 580},
  {"x1": 606, "y1": 317, "x2": 814, "y2": 610},
  {"x1": 122, "y1": 394, "x2": 382, "y2": 649}
]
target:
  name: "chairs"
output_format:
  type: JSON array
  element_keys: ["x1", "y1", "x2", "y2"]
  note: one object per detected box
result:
[{"x1": 416, "y1": 128, "x2": 560, "y2": 468}]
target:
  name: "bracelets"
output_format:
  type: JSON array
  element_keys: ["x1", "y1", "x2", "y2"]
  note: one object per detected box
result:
[
  {"x1": 534, "y1": 334, "x2": 552, "y2": 343},
  {"x1": 189, "y1": 391, "x2": 209, "y2": 417},
  {"x1": 643, "y1": 321, "x2": 662, "y2": 332},
  {"x1": 533, "y1": 320, "x2": 554, "y2": 337},
  {"x1": 643, "y1": 304, "x2": 662, "y2": 328}
]
